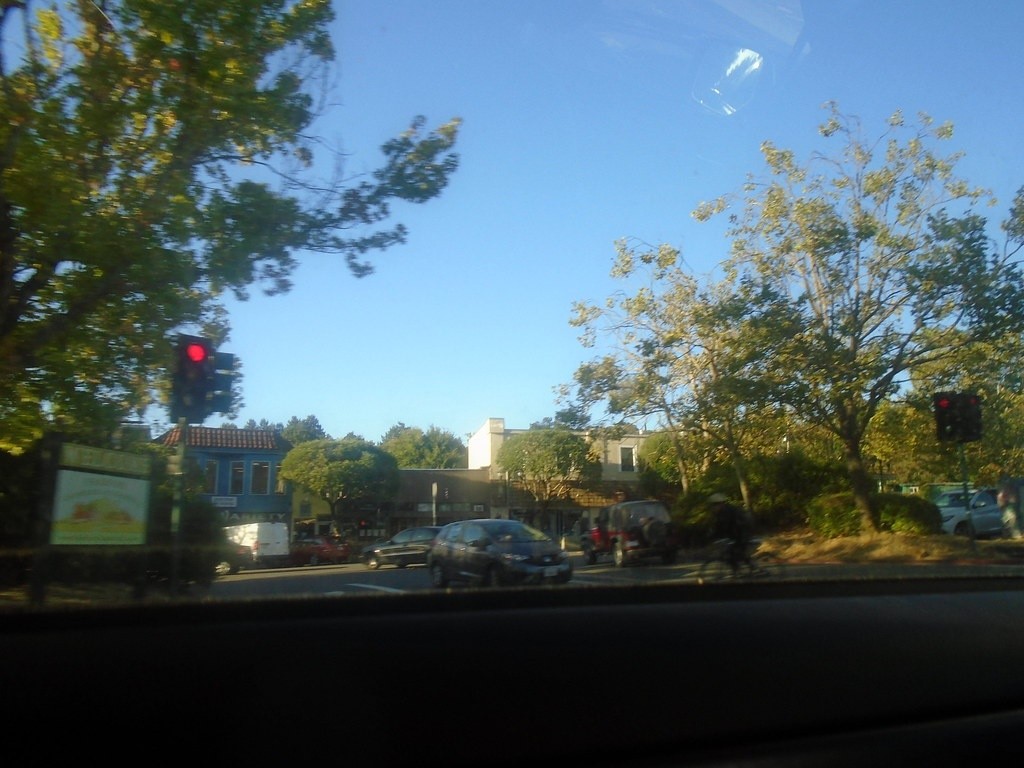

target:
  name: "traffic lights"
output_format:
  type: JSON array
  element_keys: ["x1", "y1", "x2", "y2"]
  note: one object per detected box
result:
[
  {"x1": 958, "y1": 395, "x2": 981, "y2": 442},
  {"x1": 168, "y1": 333, "x2": 211, "y2": 425},
  {"x1": 207, "y1": 352, "x2": 234, "y2": 414},
  {"x1": 933, "y1": 391, "x2": 961, "y2": 442}
]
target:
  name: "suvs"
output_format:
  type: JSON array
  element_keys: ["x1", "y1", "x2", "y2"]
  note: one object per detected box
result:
[{"x1": 580, "y1": 499, "x2": 674, "y2": 567}]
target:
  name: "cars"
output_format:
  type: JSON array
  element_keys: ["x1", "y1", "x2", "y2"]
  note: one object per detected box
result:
[
  {"x1": 429, "y1": 519, "x2": 572, "y2": 588},
  {"x1": 358, "y1": 525, "x2": 442, "y2": 568},
  {"x1": 290, "y1": 537, "x2": 350, "y2": 566},
  {"x1": 214, "y1": 538, "x2": 254, "y2": 575},
  {"x1": 937, "y1": 489, "x2": 1004, "y2": 540}
]
